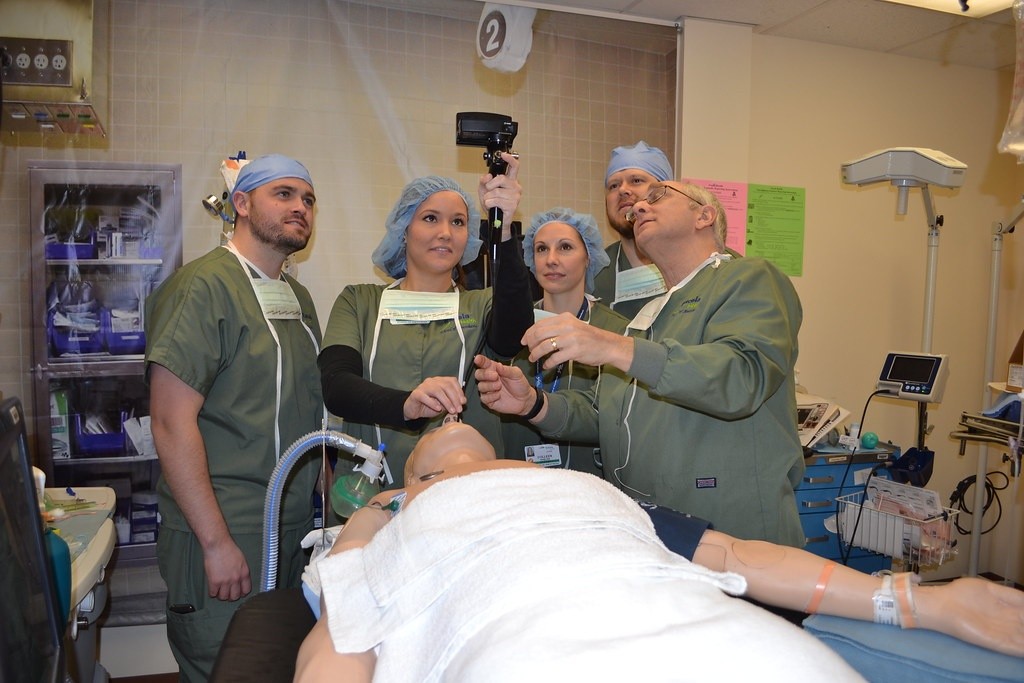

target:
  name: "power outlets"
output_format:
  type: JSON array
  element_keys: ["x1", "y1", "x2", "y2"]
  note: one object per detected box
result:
[{"x1": 0, "y1": 36, "x2": 73, "y2": 87}]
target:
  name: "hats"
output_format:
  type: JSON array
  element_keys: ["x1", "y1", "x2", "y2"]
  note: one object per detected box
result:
[
  {"x1": 229, "y1": 153, "x2": 314, "y2": 212},
  {"x1": 605, "y1": 140, "x2": 673, "y2": 187}
]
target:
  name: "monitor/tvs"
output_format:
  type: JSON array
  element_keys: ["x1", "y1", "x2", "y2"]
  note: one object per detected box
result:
[{"x1": 0, "y1": 397, "x2": 65, "y2": 683}]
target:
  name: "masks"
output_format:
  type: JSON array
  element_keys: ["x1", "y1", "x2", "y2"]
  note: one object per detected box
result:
[
  {"x1": 623, "y1": 251, "x2": 732, "y2": 342},
  {"x1": 609, "y1": 240, "x2": 668, "y2": 309},
  {"x1": 242, "y1": 268, "x2": 309, "y2": 328},
  {"x1": 533, "y1": 292, "x2": 602, "y2": 324},
  {"x1": 374, "y1": 276, "x2": 465, "y2": 342}
]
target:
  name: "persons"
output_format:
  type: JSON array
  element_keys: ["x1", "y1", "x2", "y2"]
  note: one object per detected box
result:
[
  {"x1": 526, "y1": 447, "x2": 534, "y2": 457},
  {"x1": 145, "y1": 141, "x2": 806, "y2": 683},
  {"x1": 289, "y1": 413, "x2": 1023, "y2": 683}
]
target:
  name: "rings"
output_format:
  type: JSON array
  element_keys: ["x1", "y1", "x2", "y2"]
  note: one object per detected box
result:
[{"x1": 550, "y1": 337, "x2": 558, "y2": 350}]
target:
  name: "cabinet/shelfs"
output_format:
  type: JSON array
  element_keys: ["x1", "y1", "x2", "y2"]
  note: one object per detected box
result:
[
  {"x1": 16, "y1": 159, "x2": 183, "y2": 564},
  {"x1": 790, "y1": 438, "x2": 900, "y2": 577}
]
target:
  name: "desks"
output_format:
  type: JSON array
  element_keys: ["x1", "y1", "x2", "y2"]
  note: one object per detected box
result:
[{"x1": 44, "y1": 484, "x2": 121, "y2": 610}]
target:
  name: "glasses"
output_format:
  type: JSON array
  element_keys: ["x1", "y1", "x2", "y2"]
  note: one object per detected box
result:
[{"x1": 625, "y1": 185, "x2": 703, "y2": 225}]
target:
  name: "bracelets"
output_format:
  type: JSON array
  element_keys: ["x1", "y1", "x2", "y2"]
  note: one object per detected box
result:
[
  {"x1": 891, "y1": 571, "x2": 918, "y2": 630},
  {"x1": 521, "y1": 386, "x2": 544, "y2": 419},
  {"x1": 803, "y1": 560, "x2": 834, "y2": 613},
  {"x1": 873, "y1": 575, "x2": 898, "y2": 626}
]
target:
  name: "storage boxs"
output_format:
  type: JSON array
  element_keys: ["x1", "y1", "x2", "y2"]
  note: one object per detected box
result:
[
  {"x1": 45, "y1": 216, "x2": 147, "y2": 351},
  {"x1": 43, "y1": 387, "x2": 128, "y2": 462},
  {"x1": 78, "y1": 472, "x2": 159, "y2": 546}
]
